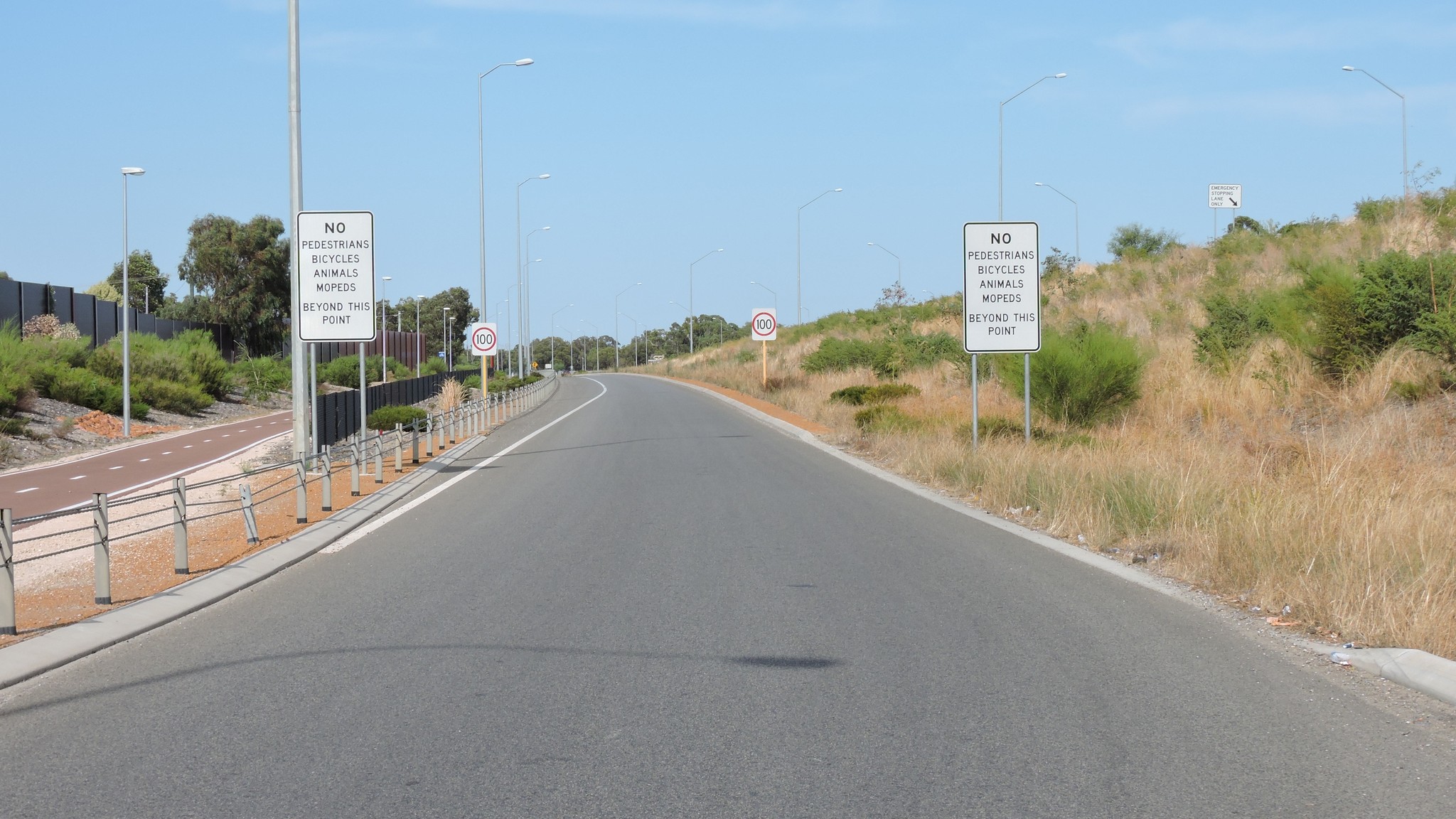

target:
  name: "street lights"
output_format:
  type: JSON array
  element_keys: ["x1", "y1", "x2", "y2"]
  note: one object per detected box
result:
[
  {"x1": 382, "y1": 276, "x2": 392, "y2": 384},
  {"x1": 513, "y1": 329, "x2": 534, "y2": 372},
  {"x1": 800, "y1": 307, "x2": 810, "y2": 322},
  {"x1": 615, "y1": 283, "x2": 649, "y2": 369},
  {"x1": 868, "y1": 242, "x2": 901, "y2": 306},
  {"x1": 478, "y1": 58, "x2": 534, "y2": 398},
  {"x1": 120, "y1": 167, "x2": 146, "y2": 436},
  {"x1": 751, "y1": 282, "x2": 777, "y2": 325},
  {"x1": 998, "y1": 73, "x2": 1066, "y2": 223},
  {"x1": 797, "y1": 188, "x2": 843, "y2": 326},
  {"x1": 551, "y1": 304, "x2": 601, "y2": 371},
  {"x1": 417, "y1": 295, "x2": 425, "y2": 378},
  {"x1": 517, "y1": 174, "x2": 551, "y2": 380},
  {"x1": 526, "y1": 226, "x2": 551, "y2": 377},
  {"x1": 523, "y1": 259, "x2": 542, "y2": 358},
  {"x1": 1035, "y1": 182, "x2": 1080, "y2": 273},
  {"x1": 444, "y1": 307, "x2": 456, "y2": 372},
  {"x1": 712, "y1": 317, "x2": 722, "y2": 344},
  {"x1": 496, "y1": 283, "x2": 522, "y2": 379},
  {"x1": 670, "y1": 301, "x2": 693, "y2": 354},
  {"x1": 1342, "y1": 65, "x2": 1408, "y2": 199},
  {"x1": 689, "y1": 248, "x2": 723, "y2": 355}
]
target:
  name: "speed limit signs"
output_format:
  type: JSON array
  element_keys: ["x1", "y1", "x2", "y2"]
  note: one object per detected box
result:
[
  {"x1": 751, "y1": 308, "x2": 777, "y2": 341},
  {"x1": 472, "y1": 322, "x2": 497, "y2": 356}
]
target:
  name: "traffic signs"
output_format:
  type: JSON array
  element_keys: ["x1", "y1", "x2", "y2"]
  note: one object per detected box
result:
[{"x1": 1208, "y1": 184, "x2": 1242, "y2": 208}]
